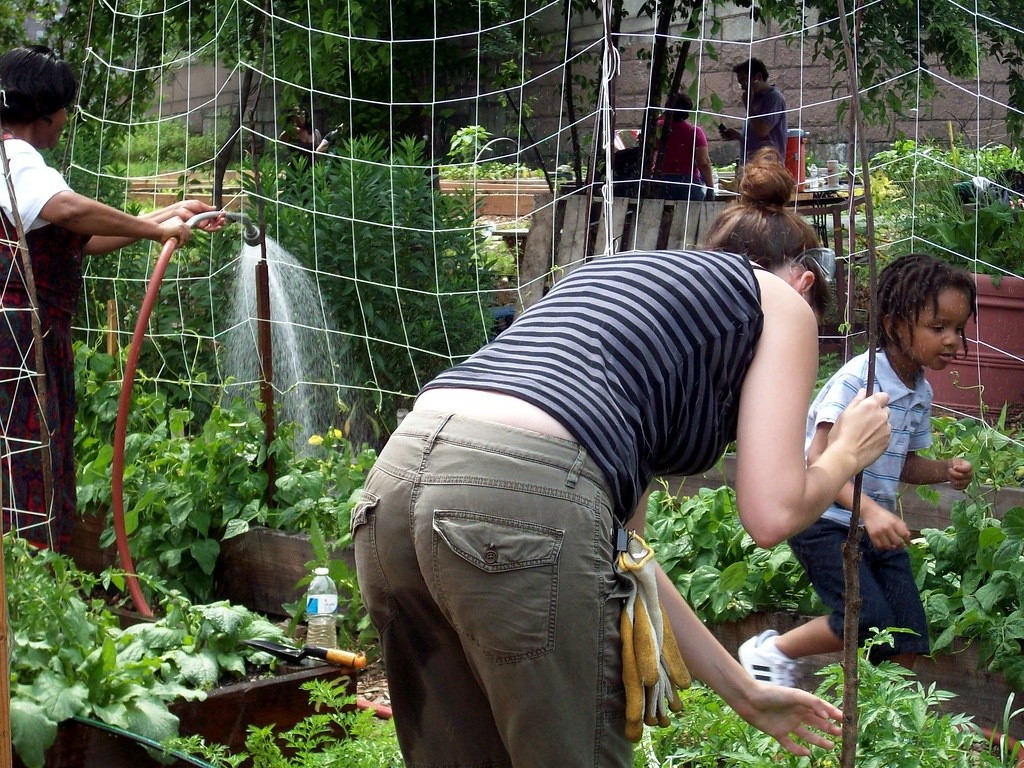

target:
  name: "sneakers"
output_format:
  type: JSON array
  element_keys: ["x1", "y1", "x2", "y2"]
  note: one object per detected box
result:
[{"x1": 738, "y1": 629, "x2": 798, "y2": 690}]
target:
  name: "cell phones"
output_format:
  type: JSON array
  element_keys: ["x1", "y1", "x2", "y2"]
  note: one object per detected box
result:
[{"x1": 718, "y1": 123, "x2": 731, "y2": 138}]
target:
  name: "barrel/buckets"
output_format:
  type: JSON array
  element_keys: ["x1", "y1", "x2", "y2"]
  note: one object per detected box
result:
[{"x1": 785, "y1": 128, "x2": 810, "y2": 192}]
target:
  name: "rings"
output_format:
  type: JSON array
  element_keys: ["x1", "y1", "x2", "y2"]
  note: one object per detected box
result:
[{"x1": 207, "y1": 224, "x2": 211, "y2": 227}]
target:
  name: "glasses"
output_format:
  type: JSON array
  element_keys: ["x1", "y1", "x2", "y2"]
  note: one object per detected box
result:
[
  {"x1": 791, "y1": 247, "x2": 836, "y2": 284},
  {"x1": 737, "y1": 80, "x2": 762, "y2": 86}
]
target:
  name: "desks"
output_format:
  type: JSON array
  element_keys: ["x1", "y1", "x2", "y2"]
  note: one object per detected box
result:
[{"x1": 719, "y1": 183, "x2": 865, "y2": 248}]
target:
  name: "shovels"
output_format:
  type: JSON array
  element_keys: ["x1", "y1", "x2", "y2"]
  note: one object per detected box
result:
[{"x1": 244, "y1": 639, "x2": 366, "y2": 669}]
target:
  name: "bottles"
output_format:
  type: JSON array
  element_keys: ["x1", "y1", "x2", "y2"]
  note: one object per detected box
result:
[
  {"x1": 307, "y1": 567, "x2": 339, "y2": 660},
  {"x1": 810, "y1": 164, "x2": 818, "y2": 188},
  {"x1": 712, "y1": 170, "x2": 719, "y2": 192}
]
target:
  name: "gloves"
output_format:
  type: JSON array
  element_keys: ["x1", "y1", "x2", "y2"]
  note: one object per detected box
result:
[{"x1": 616, "y1": 532, "x2": 694, "y2": 743}]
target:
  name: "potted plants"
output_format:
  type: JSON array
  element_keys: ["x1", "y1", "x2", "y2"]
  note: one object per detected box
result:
[{"x1": 874, "y1": 138, "x2": 1023, "y2": 432}]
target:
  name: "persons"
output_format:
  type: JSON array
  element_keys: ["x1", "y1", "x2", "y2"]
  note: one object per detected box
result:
[
  {"x1": 277, "y1": 103, "x2": 323, "y2": 207},
  {"x1": 0, "y1": 47, "x2": 227, "y2": 555},
  {"x1": 738, "y1": 252, "x2": 977, "y2": 688},
  {"x1": 719, "y1": 58, "x2": 787, "y2": 174},
  {"x1": 648, "y1": 94, "x2": 715, "y2": 201},
  {"x1": 347, "y1": 146, "x2": 891, "y2": 768},
  {"x1": 316, "y1": 122, "x2": 353, "y2": 164}
]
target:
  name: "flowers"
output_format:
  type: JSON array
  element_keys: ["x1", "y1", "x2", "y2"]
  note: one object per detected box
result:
[{"x1": 273, "y1": 429, "x2": 378, "y2": 539}]
